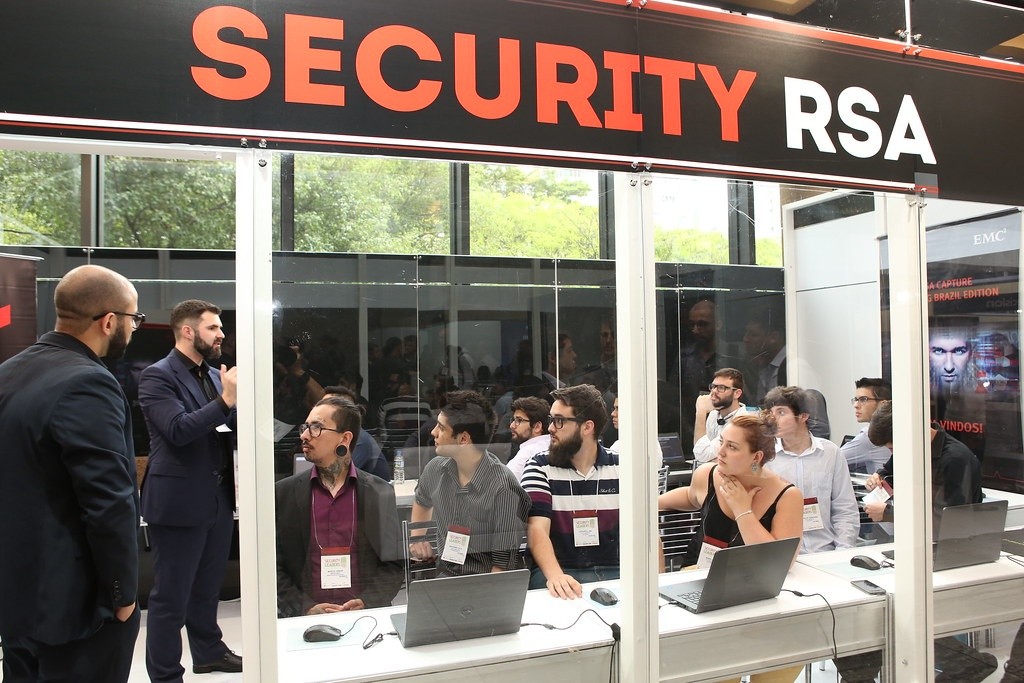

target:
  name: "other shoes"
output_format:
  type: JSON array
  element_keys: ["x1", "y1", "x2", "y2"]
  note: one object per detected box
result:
[{"x1": 935, "y1": 654, "x2": 998, "y2": 682}]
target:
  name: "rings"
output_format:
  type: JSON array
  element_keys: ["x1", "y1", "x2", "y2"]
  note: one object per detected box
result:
[{"x1": 868, "y1": 483, "x2": 873, "y2": 486}]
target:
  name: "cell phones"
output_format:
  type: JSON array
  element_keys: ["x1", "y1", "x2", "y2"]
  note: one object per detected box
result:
[{"x1": 851, "y1": 580, "x2": 886, "y2": 595}]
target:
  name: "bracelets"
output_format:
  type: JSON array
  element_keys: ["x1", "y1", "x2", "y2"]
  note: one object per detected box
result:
[{"x1": 735, "y1": 509, "x2": 752, "y2": 521}]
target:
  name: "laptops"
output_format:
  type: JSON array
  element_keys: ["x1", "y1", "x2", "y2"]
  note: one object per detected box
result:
[
  {"x1": 660, "y1": 537, "x2": 800, "y2": 613},
  {"x1": 658, "y1": 432, "x2": 698, "y2": 471},
  {"x1": 390, "y1": 569, "x2": 531, "y2": 647},
  {"x1": 882, "y1": 500, "x2": 1009, "y2": 572}
]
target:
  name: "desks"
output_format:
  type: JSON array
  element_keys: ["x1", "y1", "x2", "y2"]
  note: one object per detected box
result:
[{"x1": 277, "y1": 459, "x2": 1024, "y2": 683}]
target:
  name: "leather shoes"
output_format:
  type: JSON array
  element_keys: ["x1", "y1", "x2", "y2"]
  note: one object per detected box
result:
[{"x1": 193, "y1": 646, "x2": 242, "y2": 674}]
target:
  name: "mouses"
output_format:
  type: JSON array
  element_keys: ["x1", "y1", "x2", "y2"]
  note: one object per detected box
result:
[
  {"x1": 850, "y1": 555, "x2": 880, "y2": 570},
  {"x1": 304, "y1": 625, "x2": 341, "y2": 642},
  {"x1": 590, "y1": 588, "x2": 618, "y2": 606}
]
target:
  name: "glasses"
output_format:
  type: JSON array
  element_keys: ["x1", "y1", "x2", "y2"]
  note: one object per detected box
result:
[
  {"x1": 851, "y1": 396, "x2": 886, "y2": 406},
  {"x1": 708, "y1": 384, "x2": 737, "y2": 393},
  {"x1": 547, "y1": 415, "x2": 588, "y2": 429},
  {"x1": 389, "y1": 380, "x2": 400, "y2": 385},
  {"x1": 510, "y1": 418, "x2": 530, "y2": 426},
  {"x1": 92, "y1": 311, "x2": 145, "y2": 329},
  {"x1": 299, "y1": 424, "x2": 344, "y2": 438}
]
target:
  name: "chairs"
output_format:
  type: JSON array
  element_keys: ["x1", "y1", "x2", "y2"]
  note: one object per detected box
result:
[
  {"x1": 402, "y1": 519, "x2": 443, "y2": 603},
  {"x1": 657, "y1": 508, "x2": 702, "y2": 572},
  {"x1": 655, "y1": 465, "x2": 668, "y2": 496}
]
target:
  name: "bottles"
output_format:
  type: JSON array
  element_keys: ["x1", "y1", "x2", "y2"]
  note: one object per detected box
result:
[{"x1": 393, "y1": 451, "x2": 405, "y2": 483}]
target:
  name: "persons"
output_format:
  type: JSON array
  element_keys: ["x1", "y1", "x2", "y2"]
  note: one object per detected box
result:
[
  {"x1": 658, "y1": 411, "x2": 803, "y2": 570},
  {"x1": 929, "y1": 326, "x2": 973, "y2": 391},
  {"x1": 841, "y1": 376, "x2": 997, "y2": 683},
  {"x1": 693, "y1": 367, "x2": 746, "y2": 465},
  {"x1": 275, "y1": 388, "x2": 405, "y2": 620},
  {"x1": 0, "y1": 264, "x2": 145, "y2": 683},
  {"x1": 505, "y1": 385, "x2": 667, "y2": 599},
  {"x1": 135, "y1": 298, "x2": 243, "y2": 683},
  {"x1": 753, "y1": 384, "x2": 880, "y2": 683},
  {"x1": 672, "y1": 280, "x2": 788, "y2": 443},
  {"x1": 409, "y1": 389, "x2": 532, "y2": 580},
  {"x1": 122, "y1": 315, "x2": 617, "y2": 470}
]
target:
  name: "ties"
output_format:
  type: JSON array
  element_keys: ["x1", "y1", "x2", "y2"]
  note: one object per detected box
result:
[{"x1": 194, "y1": 367, "x2": 215, "y2": 403}]
